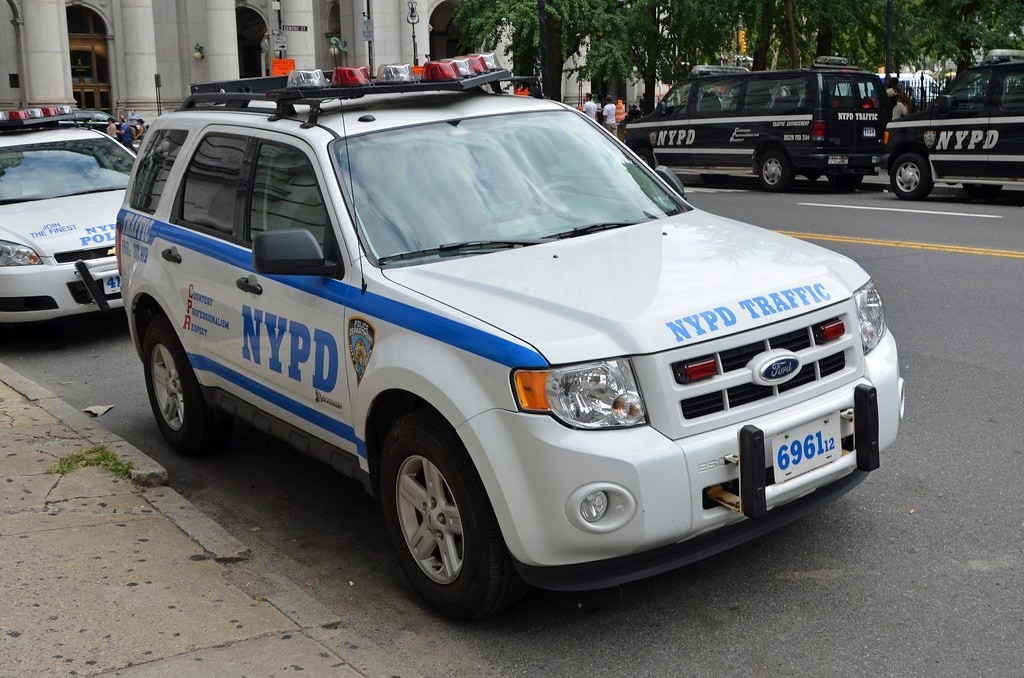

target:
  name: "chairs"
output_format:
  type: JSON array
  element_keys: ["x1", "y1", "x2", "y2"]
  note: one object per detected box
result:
[
  {"x1": 698, "y1": 91, "x2": 722, "y2": 112},
  {"x1": 746, "y1": 84, "x2": 773, "y2": 108}
]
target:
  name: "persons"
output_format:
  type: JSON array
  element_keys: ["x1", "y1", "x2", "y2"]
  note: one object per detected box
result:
[
  {"x1": 602, "y1": 95, "x2": 618, "y2": 137},
  {"x1": 107, "y1": 109, "x2": 147, "y2": 154},
  {"x1": 582, "y1": 93, "x2": 597, "y2": 121},
  {"x1": 639, "y1": 93, "x2": 644, "y2": 111},
  {"x1": 885, "y1": 88, "x2": 909, "y2": 120}
]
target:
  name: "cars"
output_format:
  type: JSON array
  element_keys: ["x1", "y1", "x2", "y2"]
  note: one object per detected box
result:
[
  {"x1": 60, "y1": 109, "x2": 141, "y2": 144},
  {"x1": 0, "y1": 103, "x2": 139, "y2": 329}
]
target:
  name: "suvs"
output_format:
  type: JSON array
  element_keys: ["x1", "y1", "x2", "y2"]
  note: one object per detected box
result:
[{"x1": 115, "y1": 50, "x2": 904, "y2": 622}]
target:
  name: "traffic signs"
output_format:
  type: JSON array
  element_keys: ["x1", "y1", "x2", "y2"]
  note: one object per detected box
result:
[{"x1": 274, "y1": 36, "x2": 288, "y2": 51}]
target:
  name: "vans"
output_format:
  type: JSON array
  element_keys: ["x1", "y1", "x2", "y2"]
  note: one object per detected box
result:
[
  {"x1": 624, "y1": 56, "x2": 891, "y2": 193},
  {"x1": 883, "y1": 47, "x2": 1024, "y2": 206}
]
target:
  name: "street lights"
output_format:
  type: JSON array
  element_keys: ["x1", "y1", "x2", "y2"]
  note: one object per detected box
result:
[{"x1": 407, "y1": 1, "x2": 419, "y2": 66}]
